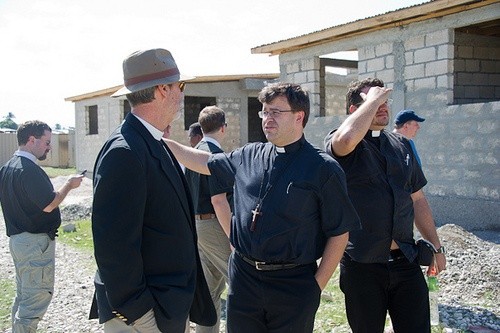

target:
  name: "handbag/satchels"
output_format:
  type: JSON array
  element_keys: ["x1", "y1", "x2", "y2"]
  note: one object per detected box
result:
[{"x1": 416, "y1": 238, "x2": 438, "y2": 266}]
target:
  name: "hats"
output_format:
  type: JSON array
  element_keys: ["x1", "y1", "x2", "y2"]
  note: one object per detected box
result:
[
  {"x1": 394, "y1": 110, "x2": 426, "y2": 125},
  {"x1": 110, "y1": 47, "x2": 196, "y2": 98}
]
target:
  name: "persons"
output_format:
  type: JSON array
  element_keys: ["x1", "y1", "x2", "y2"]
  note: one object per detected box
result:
[
  {"x1": 162, "y1": 82, "x2": 363, "y2": 333},
  {"x1": 324, "y1": 78, "x2": 446, "y2": 333},
  {"x1": 89, "y1": 48, "x2": 218, "y2": 333},
  {"x1": 189, "y1": 122, "x2": 204, "y2": 147},
  {"x1": 0, "y1": 121, "x2": 86, "y2": 333},
  {"x1": 392, "y1": 110, "x2": 425, "y2": 169},
  {"x1": 185, "y1": 105, "x2": 236, "y2": 333}
]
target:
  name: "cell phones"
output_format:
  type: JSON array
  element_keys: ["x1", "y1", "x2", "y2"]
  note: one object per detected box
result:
[{"x1": 80, "y1": 169, "x2": 87, "y2": 175}]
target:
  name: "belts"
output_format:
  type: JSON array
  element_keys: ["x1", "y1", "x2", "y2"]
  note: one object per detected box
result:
[
  {"x1": 385, "y1": 255, "x2": 404, "y2": 263},
  {"x1": 195, "y1": 213, "x2": 217, "y2": 220},
  {"x1": 234, "y1": 249, "x2": 315, "y2": 271}
]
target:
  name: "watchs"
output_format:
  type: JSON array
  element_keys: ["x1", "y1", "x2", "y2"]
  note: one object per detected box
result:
[{"x1": 435, "y1": 246, "x2": 444, "y2": 254}]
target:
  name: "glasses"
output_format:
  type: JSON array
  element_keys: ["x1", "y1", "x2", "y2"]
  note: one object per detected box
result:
[
  {"x1": 178, "y1": 81, "x2": 187, "y2": 93},
  {"x1": 353, "y1": 99, "x2": 393, "y2": 107},
  {"x1": 224, "y1": 123, "x2": 227, "y2": 128},
  {"x1": 258, "y1": 109, "x2": 297, "y2": 119}
]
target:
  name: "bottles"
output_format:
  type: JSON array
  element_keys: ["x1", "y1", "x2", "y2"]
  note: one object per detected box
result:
[{"x1": 426, "y1": 268, "x2": 439, "y2": 325}]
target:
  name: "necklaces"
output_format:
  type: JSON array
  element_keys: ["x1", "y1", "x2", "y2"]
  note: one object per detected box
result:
[{"x1": 250, "y1": 141, "x2": 307, "y2": 231}]
